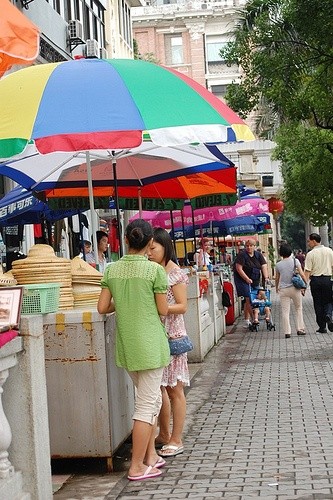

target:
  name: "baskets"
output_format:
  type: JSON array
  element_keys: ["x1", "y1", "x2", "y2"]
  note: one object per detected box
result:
[{"x1": 16, "y1": 283, "x2": 60, "y2": 315}]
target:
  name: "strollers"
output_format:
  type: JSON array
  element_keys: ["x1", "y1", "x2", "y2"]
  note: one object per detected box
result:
[{"x1": 247, "y1": 281, "x2": 276, "y2": 332}]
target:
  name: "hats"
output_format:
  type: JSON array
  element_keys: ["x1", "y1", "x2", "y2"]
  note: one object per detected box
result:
[
  {"x1": 72, "y1": 256, "x2": 103, "y2": 308},
  {"x1": 11, "y1": 245, "x2": 74, "y2": 310},
  {"x1": 245, "y1": 239, "x2": 256, "y2": 245}
]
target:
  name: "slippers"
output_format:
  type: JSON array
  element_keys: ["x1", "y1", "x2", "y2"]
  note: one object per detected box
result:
[
  {"x1": 153, "y1": 457, "x2": 167, "y2": 468},
  {"x1": 158, "y1": 445, "x2": 184, "y2": 457},
  {"x1": 127, "y1": 465, "x2": 162, "y2": 481},
  {"x1": 155, "y1": 435, "x2": 167, "y2": 447}
]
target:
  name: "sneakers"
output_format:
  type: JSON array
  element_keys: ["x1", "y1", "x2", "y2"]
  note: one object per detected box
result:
[{"x1": 245, "y1": 320, "x2": 252, "y2": 326}]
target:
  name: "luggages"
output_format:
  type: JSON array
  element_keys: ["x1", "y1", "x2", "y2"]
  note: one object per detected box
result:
[{"x1": 213, "y1": 264, "x2": 234, "y2": 326}]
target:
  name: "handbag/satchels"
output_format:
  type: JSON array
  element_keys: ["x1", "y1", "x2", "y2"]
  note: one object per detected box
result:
[
  {"x1": 222, "y1": 292, "x2": 232, "y2": 307},
  {"x1": 292, "y1": 258, "x2": 307, "y2": 289},
  {"x1": 168, "y1": 336, "x2": 193, "y2": 355},
  {"x1": 250, "y1": 267, "x2": 260, "y2": 280}
]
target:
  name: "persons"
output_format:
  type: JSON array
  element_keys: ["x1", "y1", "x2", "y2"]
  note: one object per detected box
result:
[
  {"x1": 295, "y1": 249, "x2": 305, "y2": 272},
  {"x1": 275, "y1": 244, "x2": 308, "y2": 338},
  {"x1": 146, "y1": 227, "x2": 191, "y2": 457},
  {"x1": 81, "y1": 231, "x2": 108, "y2": 275},
  {"x1": 97, "y1": 219, "x2": 171, "y2": 480},
  {"x1": 234, "y1": 238, "x2": 269, "y2": 329},
  {"x1": 301, "y1": 233, "x2": 333, "y2": 333},
  {"x1": 193, "y1": 238, "x2": 211, "y2": 265},
  {"x1": 252, "y1": 289, "x2": 270, "y2": 323},
  {"x1": 217, "y1": 245, "x2": 230, "y2": 264},
  {"x1": 78, "y1": 240, "x2": 91, "y2": 259}
]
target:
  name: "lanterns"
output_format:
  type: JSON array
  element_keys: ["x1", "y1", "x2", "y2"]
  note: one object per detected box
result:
[{"x1": 266, "y1": 198, "x2": 284, "y2": 219}]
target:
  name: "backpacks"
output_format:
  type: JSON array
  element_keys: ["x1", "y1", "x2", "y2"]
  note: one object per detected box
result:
[{"x1": 184, "y1": 252, "x2": 200, "y2": 266}]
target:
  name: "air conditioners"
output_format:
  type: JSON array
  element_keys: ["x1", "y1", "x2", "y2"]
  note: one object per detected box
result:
[
  {"x1": 99, "y1": 48, "x2": 107, "y2": 59},
  {"x1": 86, "y1": 39, "x2": 98, "y2": 58},
  {"x1": 66, "y1": 19, "x2": 83, "y2": 41}
]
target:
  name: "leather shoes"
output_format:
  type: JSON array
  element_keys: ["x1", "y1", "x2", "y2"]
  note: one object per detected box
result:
[
  {"x1": 316, "y1": 328, "x2": 326, "y2": 333},
  {"x1": 286, "y1": 334, "x2": 290, "y2": 338},
  {"x1": 325, "y1": 314, "x2": 333, "y2": 332},
  {"x1": 297, "y1": 331, "x2": 306, "y2": 335}
]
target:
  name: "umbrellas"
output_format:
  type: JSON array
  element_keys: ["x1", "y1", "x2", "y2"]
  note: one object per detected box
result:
[
  {"x1": 0, "y1": 58, "x2": 256, "y2": 272},
  {"x1": 128, "y1": 196, "x2": 269, "y2": 261},
  {"x1": 168, "y1": 213, "x2": 263, "y2": 262},
  {"x1": 0, "y1": 184, "x2": 90, "y2": 247},
  {"x1": 0, "y1": 135, "x2": 235, "y2": 257},
  {"x1": 32, "y1": 166, "x2": 238, "y2": 219}
]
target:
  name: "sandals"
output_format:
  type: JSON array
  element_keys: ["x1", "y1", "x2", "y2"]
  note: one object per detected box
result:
[
  {"x1": 253, "y1": 320, "x2": 259, "y2": 324},
  {"x1": 266, "y1": 320, "x2": 270, "y2": 323}
]
